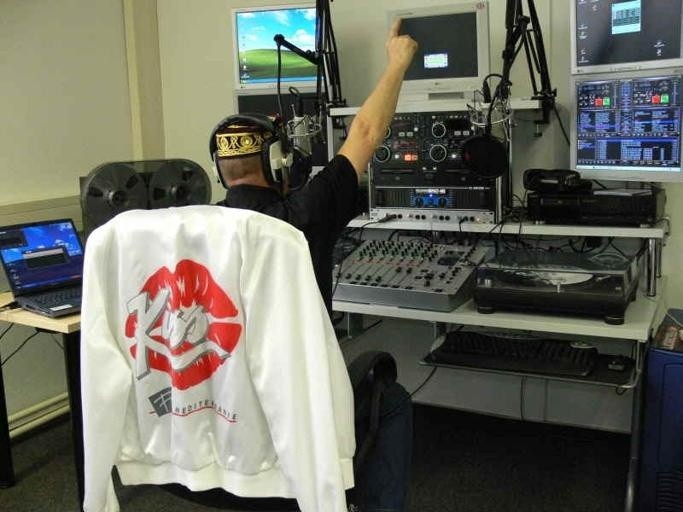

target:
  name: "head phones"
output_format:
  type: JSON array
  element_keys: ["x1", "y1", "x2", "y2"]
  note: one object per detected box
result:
[{"x1": 209, "y1": 112, "x2": 312, "y2": 191}]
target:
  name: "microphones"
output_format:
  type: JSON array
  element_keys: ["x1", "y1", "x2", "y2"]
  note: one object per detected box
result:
[
  {"x1": 288, "y1": 97, "x2": 311, "y2": 158},
  {"x1": 476, "y1": 79, "x2": 508, "y2": 146}
]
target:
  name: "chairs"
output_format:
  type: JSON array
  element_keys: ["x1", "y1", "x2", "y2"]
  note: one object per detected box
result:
[{"x1": 76, "y1": 203, "x2": 417, "y2": 511}]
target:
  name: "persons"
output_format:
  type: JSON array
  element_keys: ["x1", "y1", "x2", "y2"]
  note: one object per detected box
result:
[{"x1": 210, "y1": 17, "x2": 417, "y2": 512}]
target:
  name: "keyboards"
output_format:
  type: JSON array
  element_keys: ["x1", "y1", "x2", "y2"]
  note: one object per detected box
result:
[{"x1": 418, "y1": 326, "x2": 599, "y2": 378}]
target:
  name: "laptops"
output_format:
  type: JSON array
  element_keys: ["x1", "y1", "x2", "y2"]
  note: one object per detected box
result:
[{"x1": 0, "y1": 218, "x2": 89, "y2": 318}]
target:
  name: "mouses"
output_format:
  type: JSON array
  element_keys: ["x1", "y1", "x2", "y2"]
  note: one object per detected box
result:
[{"x1": 605, "y1": 351, "x2": 628, "y2": 375}]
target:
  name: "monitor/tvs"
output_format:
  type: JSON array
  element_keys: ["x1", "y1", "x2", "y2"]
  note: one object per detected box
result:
[
  {"x1": 568, "y1": 0, "x2": 683, "y2": 76},
  {"x1": 569, "y1": 68, "x2": 683, "y2": 185},
  {"x1": 386, "y1": 1, "x2": 492, "y2": 100},
  {"x1": 230, "y1": 4, "x2": 328, "y2": 96}
]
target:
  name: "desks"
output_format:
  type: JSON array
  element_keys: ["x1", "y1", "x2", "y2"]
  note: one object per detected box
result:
[
  {"x1": 324, "y1": 98, "x2": 670, "y2": 512},
  {"x1": 0, "y1": 290, "x2": 86, "y2": 510}
]
